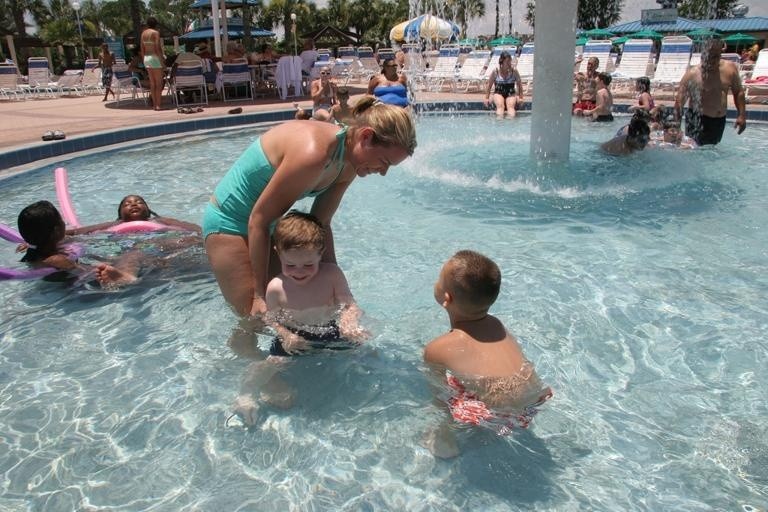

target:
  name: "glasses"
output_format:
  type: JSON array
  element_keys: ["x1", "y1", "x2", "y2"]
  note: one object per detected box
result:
[
  {"x1": 385, "y1": 64, "x2": 398, "y2": 68},
  {"x1": 320, "y1": 70, "x2": 332, "y2": 76}
]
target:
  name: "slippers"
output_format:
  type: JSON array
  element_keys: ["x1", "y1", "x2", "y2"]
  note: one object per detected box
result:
[
  {"x1": 228, "y1": 107, "x2": 243, "y2": 115},
  {"x1": 42, "y1": 129, "x2": 54, "y2": 140},
  {"x1": 54, "y1": 129, "x2": 66, "y2": 140}
]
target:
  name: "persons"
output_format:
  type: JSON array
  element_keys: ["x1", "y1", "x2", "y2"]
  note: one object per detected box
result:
[
  {"x1": 483, "y1": 50, "x2": 524, "y2": 119},
  {"x1": 168, "y1": 43, "x2": 204, "y2": 105},
  {"x1": 139, "y1": 17, "x2": 169, "y2": 111},
  {"x1": 674, "y1": 40, "x2": 747, "y2": 146},
  {"x1": 195, "y1": 42, "x2": 217, "y2": 84},
  {"x1": 327, "y1": 86, "x2": 353, "y2": 123},
  {"x1": 572, "y1": 53, "x2": 599, "y2": 117},
  {"x1": 601, "y1": 120, "x2": 650, "y2": 157},
  {"x1": 300, "y1": 37, "x2": 318, "y2": 76},
  {"x1": 656, "y1": 120, "x2": 697, "y2": 151},
  {"x1": 367, "y1": 57, "x2": 416, "y2": 111},
  {"x1": 128, "y1": 47, "x2": 153, "y2": 107},
  {"x1": 740, "y1": 44, "x2": 760, "y2": 64},
  {"x1": 627, "y1": 76, "x2": 655, "y2": 114},
  {"x1": 583, "y1": 71, "x2": 614, "y2": 122},
  {"x1": 91, "y1": 43, "x2": 116, "y2": 102},
  {"x1": 292, "y1": 107, "x2": 334, "y2": 122},
  {"x1": 15, "y1": 194, "x2": 203, "y2": 288},
  {"x1": 422, "y1": 247, "x2": 554, "y2": 458},
  {"x1": 17, "y1": 199, "x2": 87, "y2": 271},
  {"x1": 310, "y1": 66, "x2": 338, "y2": 120},
  {"x1": 256, "y1": 43, "x2": 277, "y2": 64},
  {"x1": 261, "y1": 211, "x2": 370, "y2": 365},
  {"x1": 198, "y1": 96, "x2": 417, "y2": 356},
  {"x1": 220, "y1": 42, "x2": 246, "y2": 64},
  {"x1": 613, "y1": 107, "x2": 664, "y2": 136}
]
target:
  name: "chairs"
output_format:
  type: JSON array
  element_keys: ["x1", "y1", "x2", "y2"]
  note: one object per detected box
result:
[
  {"x1": 417, "y1": 43, "x2": 462, "y2": 90},
  {"x1": 689, "y1": 51, "x2": 742, "y2": 68},
  {"x1": 357, "y1": 46, "x2": 376, "y2": 66},
  {"x1": 402, "y1": 42, "x2": 418, "y2": 53},
  {"x1": 610, "y1": 38, "x2": 653, "y2": 91},
  {"x1": 112, "y1": 56, "x2": 150, "y2": 106},
  {"x1": 302, "y1": 50, "x2": 317, "y2": 95},
  {"x1": 337, "y1": 46, "x2": 356, "y2": 61},
  {"x1": 273, "y1": 55, "x2": 301, "y2": 100},
  {"x1": 743, "y1": 47, "x2": 768, "y2": 101},
  {"x1": 472, "y1": 44, "x2": 516, "y2": 92},
  {"x1": 575, "y1": 38, "x2": 615, "y2": 73},
  {"x1": 1, "y1": 60, "x2": 18, "y2": 101},
  {"x1": 649, "y1": 33, "x2": 694, "y2": 90},
  {"x1": 214, "y1": 56, "x2": 255, "y2": 103},
  {"x1": 377, "y1": 48, "x2": 394, "y2": 63},
  {"x1": 172, "y1": 56, "x2": 206, "y2": 106},
  {"x1": 44, "y1": 68, "x2": 83, "y2": 98},
  {"x1": 316, "y1": 47, "x2": 330, "y2": 63},
  {"x1": 17, "y1": 56, "x2": 50, "y2": 100},
  {"x1": 514, "y1": 41, "x2": 537, "y2": 95},
  {"x1": 79, "y1": 57, "x2": 104, "y2": 91},
  {"x1": 456, "y1": 49, "x2": 492, "y2": 92}
]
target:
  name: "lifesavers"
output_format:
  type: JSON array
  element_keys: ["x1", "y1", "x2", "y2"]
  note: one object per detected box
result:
[
  {"x1": 650, "y1": 129, "x2": 698, "y2": 152},
  {"x1": 88, "y1": 218, "x2": 184, "y2": 244},
  {"x1": 266, "y1": 320, "x2": 363, "y2": 356},
  {"x1": 442, "y1": 370, "x2": 555, "y2": 436},
  {"x1": 1, "y1": 225, "x2": 87, "y2": 281}
]
